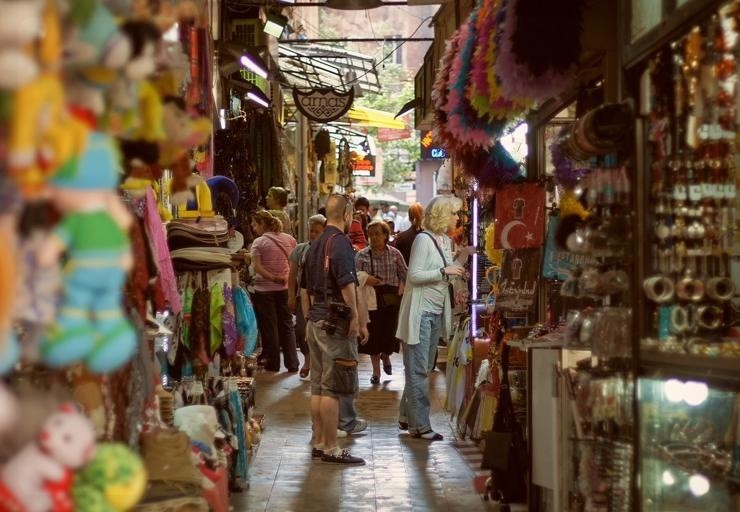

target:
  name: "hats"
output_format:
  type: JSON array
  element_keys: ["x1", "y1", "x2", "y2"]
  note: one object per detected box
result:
[
  {"x1": 166, "y1": 179, "x2": 235, "y2": 273},
  {"x1": 144, "y1": 402, "x2": 222, "y2": 487}
]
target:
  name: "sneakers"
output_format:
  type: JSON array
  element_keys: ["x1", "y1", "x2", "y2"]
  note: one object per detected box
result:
[
  {"x1": 322, "y1": 451, "x2": 365, "y2": 467},
  {"x1": 311, "y1": 447, "x2": 326, "y2": 459},
  {"x1": 339, "y1": 418, "x2": 367, "y2": 434}
]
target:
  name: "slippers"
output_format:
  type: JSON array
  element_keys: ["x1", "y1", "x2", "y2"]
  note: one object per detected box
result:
[{"x1": 300, "y1": 364, "x2": 310, "y2": 379}]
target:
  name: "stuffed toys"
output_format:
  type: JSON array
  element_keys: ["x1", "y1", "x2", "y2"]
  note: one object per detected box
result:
[{"x1": 1, "y1": 0, "x2": 192, "y2": 511}]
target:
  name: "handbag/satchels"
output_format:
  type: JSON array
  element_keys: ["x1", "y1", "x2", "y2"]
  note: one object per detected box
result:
[
  {"x1": 449, "y1": 282, "x2": 455, "y2": 310},
  {"x1": 380, "y1": 290, "x2": 400, "y2": 309},
  {"x1": 319, "y1": 302, "x2": 353, "y2": 337},
  {"x1": 480, "y1": 424, "x2": 528, "y2": 502}
]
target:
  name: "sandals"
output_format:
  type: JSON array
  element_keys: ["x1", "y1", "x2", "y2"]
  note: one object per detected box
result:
[
  {"x1": 411, "y1": 429, "x2": 445, "y2": 440},
  {"x1": 397, "y1": 417, "x2": 409, "y2": 431}
]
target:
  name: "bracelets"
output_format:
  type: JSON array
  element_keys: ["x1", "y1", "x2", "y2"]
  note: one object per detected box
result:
[
  {"x1": 644, "y1": 269, "x2": 737, "y2": 338},
  {"x1": 653, "y1": 409, "x2": 734, "y2": 480}
]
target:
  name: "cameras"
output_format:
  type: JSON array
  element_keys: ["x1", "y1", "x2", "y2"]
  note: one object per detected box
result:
[{"x1": 322, "y1": 302, "x2": 351, "y2": 334}]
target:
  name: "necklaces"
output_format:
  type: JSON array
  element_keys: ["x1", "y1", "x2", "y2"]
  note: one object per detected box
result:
[{"x1": 642, "y1": 9, "x2": 735, "y2": 262}]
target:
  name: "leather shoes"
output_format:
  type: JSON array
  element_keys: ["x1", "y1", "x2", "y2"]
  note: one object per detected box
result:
[
  {"x1": 380, "y1": 353, "x2": 393, "y2": 375},
  {"x1": 371, "y1": 375, "x2": 381, "y2": 385}
]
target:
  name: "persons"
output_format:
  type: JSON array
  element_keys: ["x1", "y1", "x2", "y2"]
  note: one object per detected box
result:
[
  {"x1": 256, "y1": 182, "x2": 297, "y2": 241},
  {"x1": 285, "y1": 213, "x2": 329, "y2": 379},
  {"x1": 313, "y1": 191, "x2": 429, "y2": 267},
  {"x1": 326, "y1": 270, "x2": 365, "y2": 436},
  {"x1": 355, "y1": 217, "x2": 409, "y2": 386},
  {"x1": 246, "y1": 210, "x2": 299, "y2": 372},
  {"x1": 299, "y1": 190, "x2": 367, "y2": 467},
  {"x1": 392, "y1": 194, "x2": 478, "y2": 442}
]
